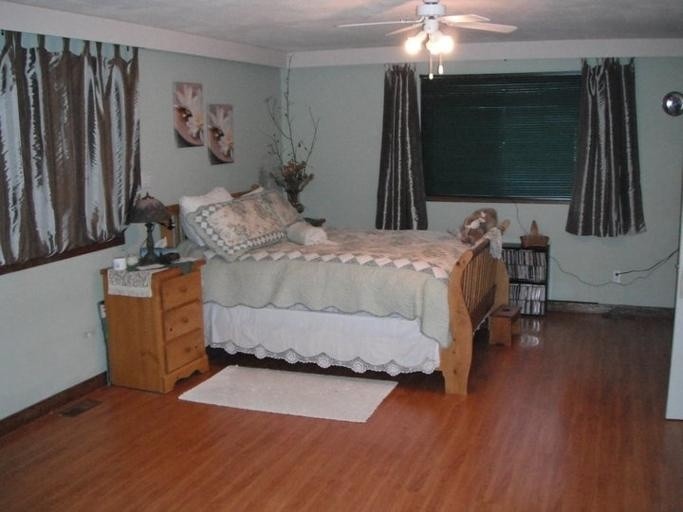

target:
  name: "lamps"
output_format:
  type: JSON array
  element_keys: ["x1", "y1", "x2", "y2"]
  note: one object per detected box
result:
[{"x1": 126, "y1": 193, "x2": 172, "y2": 270}]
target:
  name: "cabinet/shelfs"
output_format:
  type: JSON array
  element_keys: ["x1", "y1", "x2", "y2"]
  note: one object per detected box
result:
[{"x1": 502, "y1": 244, "x2": 548, "y2": 318}]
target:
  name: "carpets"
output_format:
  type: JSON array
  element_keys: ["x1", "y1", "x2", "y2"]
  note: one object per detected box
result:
[{"x1": 178, "y1": 363, "x2": 399, "y2": 423}]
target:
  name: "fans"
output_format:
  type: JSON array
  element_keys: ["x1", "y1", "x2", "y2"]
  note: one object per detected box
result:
[{"x1": 336, "y1": 0, "x2": 517, "y2": 36}]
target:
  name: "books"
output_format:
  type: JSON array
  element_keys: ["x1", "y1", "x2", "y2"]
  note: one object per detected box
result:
[{"x1": 500, "y1": 249, "x2": 547, "y2": 316}]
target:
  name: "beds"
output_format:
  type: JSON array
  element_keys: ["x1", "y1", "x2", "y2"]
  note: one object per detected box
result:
[{"x1": 160, "y1": 184, "x2": 512, "y2": 395}]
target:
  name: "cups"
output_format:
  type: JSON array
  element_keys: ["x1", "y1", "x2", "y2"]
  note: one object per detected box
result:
[{"x1": 113, "y1": 257, "x2": 126, "y2": 271}]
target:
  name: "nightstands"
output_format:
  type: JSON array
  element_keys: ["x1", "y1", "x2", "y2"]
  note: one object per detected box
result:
[{"x1": 100, "y1": 255, "x2": 211, "y2": 394}]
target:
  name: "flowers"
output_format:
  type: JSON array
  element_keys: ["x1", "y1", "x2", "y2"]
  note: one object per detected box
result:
[{"x1": 265, "y1": 49, "x2": 320, "y2": 212}]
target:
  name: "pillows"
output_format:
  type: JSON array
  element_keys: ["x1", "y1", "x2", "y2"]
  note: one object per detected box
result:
[{"x1": 178, "y1": 187, "x2": 337, "y2": 264}]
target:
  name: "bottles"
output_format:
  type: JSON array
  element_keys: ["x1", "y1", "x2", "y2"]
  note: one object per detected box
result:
[{"x1": 127, "y1": 253, "x2": 138, "y2": 267}]
